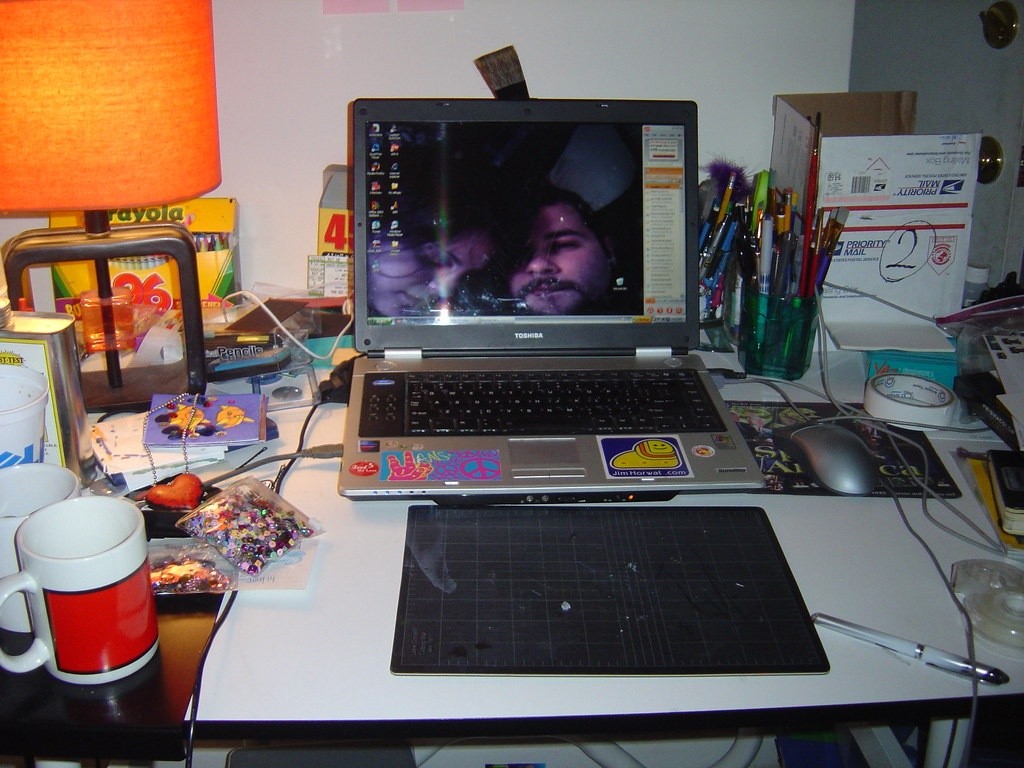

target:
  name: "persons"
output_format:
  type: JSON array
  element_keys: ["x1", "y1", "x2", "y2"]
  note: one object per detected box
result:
[{"x1": 368, "y1": 144, "x2": 614, "y2": 316}]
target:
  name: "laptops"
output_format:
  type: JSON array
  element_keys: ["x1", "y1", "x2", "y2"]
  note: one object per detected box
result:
[{"x1": 337, "y1": 98, "x2": 765, "y2": 501}]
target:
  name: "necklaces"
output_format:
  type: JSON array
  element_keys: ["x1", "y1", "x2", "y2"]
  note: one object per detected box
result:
[{"x1": 143, "y1": 392, "x2": 203, "y2": 510}]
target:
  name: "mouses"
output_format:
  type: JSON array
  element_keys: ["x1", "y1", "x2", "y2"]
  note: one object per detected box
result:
[{"x1": 772, "y1": 419, "x2": 878, "y2": 496}]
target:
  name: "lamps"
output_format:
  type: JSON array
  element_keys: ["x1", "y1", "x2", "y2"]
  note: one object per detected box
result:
[{"x1": 0, "y1": 0, "x2": 223, "y2": 412}]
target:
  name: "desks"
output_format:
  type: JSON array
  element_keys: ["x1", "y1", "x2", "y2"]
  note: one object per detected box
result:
[{"x1": 0, "y1": 297, "x2": 1024, "y2": 768}]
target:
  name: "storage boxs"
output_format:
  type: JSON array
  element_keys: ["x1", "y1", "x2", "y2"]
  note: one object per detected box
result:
[{"x1": 770, "y1": 90, "x2": 984, "y2": 351}]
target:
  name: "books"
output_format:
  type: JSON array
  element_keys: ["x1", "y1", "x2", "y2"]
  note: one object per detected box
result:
[{"x1": 961, "y1": 450, "x2": 1024, "y2": 557}]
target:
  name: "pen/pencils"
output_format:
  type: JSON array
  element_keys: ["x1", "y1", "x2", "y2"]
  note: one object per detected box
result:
[
  {"x1": 700, "y1": 169, "x2": 850, "y2": 381},
  {"x1": 809, "y1": 610, "x2": 1011, "y2": 687}
]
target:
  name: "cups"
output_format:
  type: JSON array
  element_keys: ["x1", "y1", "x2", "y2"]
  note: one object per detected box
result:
[
  {"x1": 0, "y1": 463, "x2": 82, "y2": 580},
  {"x1": 738, "y1": 278, "x2": 820, "y2": 381},
  {"x1": 700, "y1": 280, "x2": 728, "y2": 328},
  {"x1": 0, "y1": 495, "x2": 159, "y2": 684},
  {"x1": 0, "y1": 364, "x2": 48, "y2": 469},
  {"x1": 722, "y1": 251, "x2": 757, "y2": 346}
]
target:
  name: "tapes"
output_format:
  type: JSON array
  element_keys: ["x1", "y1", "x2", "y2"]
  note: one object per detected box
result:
[
  {"x1": 949, "y1": 558, "x2": 1024, "y2": 661},
  {"x1": 863, "y1": 371, "x2": 959, "y2": 433}
]
